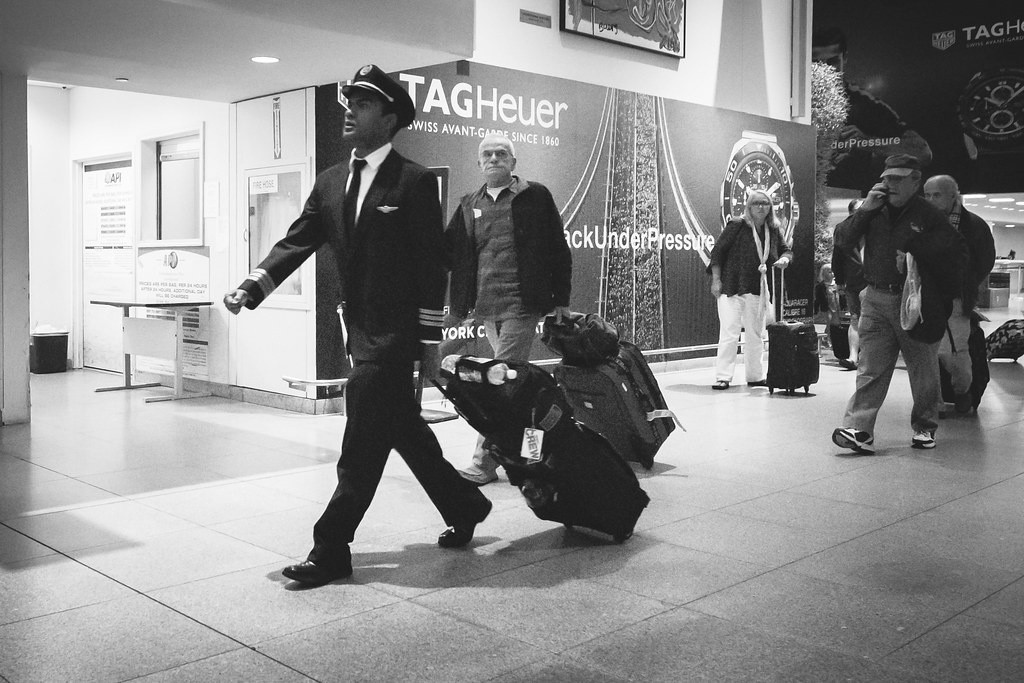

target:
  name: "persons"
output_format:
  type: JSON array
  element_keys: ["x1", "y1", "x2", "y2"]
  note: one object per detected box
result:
[
  {"x1": 446, "y1": 134, "x2": 572, "y2": 485},
  {"x1": 705, "y1": 190, "x2": 794, "y2": 390},
  {"x1": 828, "y1": 197, "x2": 864, "y2": 369},
  {"x1": 831, "y1": 153, "x2": 970, "y2": 455},
  {"x1": 924, "y1": 174, "x2": 996, "y2": 415},
  {"x1": 222, "y1": 65, "x2": 493, "y2": 585}
]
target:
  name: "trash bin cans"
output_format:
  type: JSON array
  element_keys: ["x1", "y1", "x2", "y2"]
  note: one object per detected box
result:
[{"x1": 31, "y1": 330, "x2": 70, "y2": 374}]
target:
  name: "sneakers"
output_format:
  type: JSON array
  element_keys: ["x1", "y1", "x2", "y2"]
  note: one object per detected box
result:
[
  {"x1": 832, "y1": 427, "x2": 875, "y2": 454},
  {"x1": 912, "y1": 430, "x2": 937, "y2": 448}
]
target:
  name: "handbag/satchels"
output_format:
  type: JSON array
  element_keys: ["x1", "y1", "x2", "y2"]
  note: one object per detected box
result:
[
  {"x1": 542, "y1": 311, "x2": 619, "y2": 365},
  {"x1": 440, "y1": 354, "x2": 573, "y2": 446}
]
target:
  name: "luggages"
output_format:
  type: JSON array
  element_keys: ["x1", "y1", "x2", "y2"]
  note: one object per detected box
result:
[
  {"x1": 421, "y1": 366, "x2": 650, "y2": 542},
  {"x1": 553, "y1": 313, "x2": 687, "y2": 471},
  {"x1": 766, "y1": 264, "x2": 821, "y2": 394},
  {"x1": 939, "y1": 313, "x2": 990, "y2": 411},
  {"x1": 986, "y1": 319, "x2": 1024, "y2": 362},
  {"x1": 830, "y1": 289, "x2": 861, "y2": 358}
]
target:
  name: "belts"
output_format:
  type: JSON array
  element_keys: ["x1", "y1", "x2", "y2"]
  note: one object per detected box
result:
[{"x1": 869, "y1": 282, "x2": 902, "y2": 293}]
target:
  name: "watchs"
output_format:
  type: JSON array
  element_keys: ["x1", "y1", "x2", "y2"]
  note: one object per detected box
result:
[{"x1": 719, "y1": 130, "x2": 800, "y2": 249}]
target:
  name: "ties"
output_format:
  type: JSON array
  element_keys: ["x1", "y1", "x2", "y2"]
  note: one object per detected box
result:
[{"x1": 344, "y1": 159, "x2": 367, "y2": 240}]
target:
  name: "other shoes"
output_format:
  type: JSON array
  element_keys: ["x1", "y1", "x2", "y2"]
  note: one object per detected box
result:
[
  {"x1": 954, "y1": 388, "x2": 971, "y2": 413},
  {"x1": 459, "y1": 466, "x2": 498, "y2": 484}
]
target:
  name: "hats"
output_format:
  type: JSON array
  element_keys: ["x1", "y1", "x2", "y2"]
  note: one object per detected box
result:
[
  {"x1": 880, "y1": 153, "x2": 920, "y2": 177},
  {"x1": 342, "y1": 63, "x2": 415, "y2": 127}
]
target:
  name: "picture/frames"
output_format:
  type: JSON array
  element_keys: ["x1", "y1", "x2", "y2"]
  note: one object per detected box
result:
[{"x1": 558, "y1": 0, "x2": 686, "y2": 60}]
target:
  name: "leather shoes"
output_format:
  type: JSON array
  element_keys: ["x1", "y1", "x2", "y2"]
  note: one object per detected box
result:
[
  {"x1": 282, "y1": 559, "x2": 353, "y2": 584},
  {"x1": 437, "y1": 498, "x2": 492, "y2": 544}
]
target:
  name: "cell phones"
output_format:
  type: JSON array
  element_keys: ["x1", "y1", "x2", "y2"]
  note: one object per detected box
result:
[{"x1": 880, "y1": 187, "x2": 889, "y2": 206}]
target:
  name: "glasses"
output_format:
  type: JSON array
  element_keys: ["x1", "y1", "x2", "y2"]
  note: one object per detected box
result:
[{"x1": 751, "y1": 201, "x2": 770, "y2": 207}]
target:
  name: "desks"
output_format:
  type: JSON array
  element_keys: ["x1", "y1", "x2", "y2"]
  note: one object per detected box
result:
[{"x1": 90, "y1": 300, "x2": 215, "y2": 404}]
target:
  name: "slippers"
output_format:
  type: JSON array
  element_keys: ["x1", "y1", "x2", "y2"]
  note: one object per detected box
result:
[
  {"x1": 748, "y1": 379, "x2": 767, "y2": 386},
  {"x1": 839, "y1": 358, "x2": 858, "y2": 370},
  {"x1": 712, "y1": 379, "x2": 730, "y2": 389}
]
